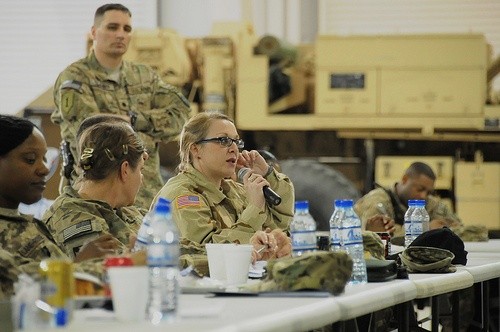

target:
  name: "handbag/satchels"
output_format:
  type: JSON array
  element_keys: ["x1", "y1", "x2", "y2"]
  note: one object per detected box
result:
[{"x1": 364, "y1": 259, "x2": 397, "y2": 282}]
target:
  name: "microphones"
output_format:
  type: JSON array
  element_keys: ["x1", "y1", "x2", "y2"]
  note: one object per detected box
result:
[{"x1": 238, "y1": 168, "x2": 282, "y2": 206}]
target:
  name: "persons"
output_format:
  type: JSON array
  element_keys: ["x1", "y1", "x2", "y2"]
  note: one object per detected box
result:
[
  {"x1": 74, "y1": 114, "x2": 293, "y2": 261},
  {"x1": 49, "y1": 3, "x2": 191, "y2": 217},
  {"x1": 352, "y1": 160, "x2": 478, "y2": 332},
  {"x1": 148, "y1": 111, "x2": 297, "y2": 258},
  {"x1": 256, "y1": 149, "x2": 397, "y2": 241},
  {"x1": 1, "y1": 114, "x2": 116, "y2": 296},
  {"x1": 39, "y1": 122, "x2": 279, "y2": 278}
]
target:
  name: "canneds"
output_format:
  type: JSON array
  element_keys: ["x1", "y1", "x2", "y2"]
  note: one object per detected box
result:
[
  {"x1": 316, "y1": 235, "x2": 329, "y2": 251},
  {"x1": 376, "y1": 232, "x2": 391, "y2": 259},
  {"x1": 40, "y1": 256, "x2": 74, "y2": 317},
  {"x1": 101, "y1": 254, "x2": 133, "y2": 297}
]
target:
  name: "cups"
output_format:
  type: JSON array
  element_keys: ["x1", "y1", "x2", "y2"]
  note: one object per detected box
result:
[
  {"x1": 205, "y1": 243, "x2": 227, "y2": 281},
  {"x1": 108, "y1": 265, "x2": 149, "y2": 320},
  {"x1": 224, "y1": 244, "x2": 253, "y2": 285},
  {"x1": 11, "y1": 282, "x2": 39, "y2": 332}
]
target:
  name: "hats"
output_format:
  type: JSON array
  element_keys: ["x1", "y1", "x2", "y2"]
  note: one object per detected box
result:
[
  {"x1": 387, "y1": 226, "x2": 468, "y2": 266},
  {"x1": 247, "y1": 251, "x2": 354, "y2": 296},
  {"x1": 398, "y1": 247, "x2": 457, "y2": 273},
  {"x1": 320, "y1": 230, "x2": 385, "y2": 260}
]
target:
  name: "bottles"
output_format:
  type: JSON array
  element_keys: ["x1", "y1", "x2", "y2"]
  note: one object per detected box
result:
[
  {"x1": 149, "y1": 198, "x2": 180, "y2": 315},
  {"x1": 290, "y1": 201, "x2": 318, "y2": 257},
  {"x1": 403, "y1": 200, "x2": 430, "y2": 247},
  {"x1": 341, "y1": 200, "x2": 368, "y2": 285},
  {"x1": 330, "y1": 200, "x2": 343, "y2": 252}
]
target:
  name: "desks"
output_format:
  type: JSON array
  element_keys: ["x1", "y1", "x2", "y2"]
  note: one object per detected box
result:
[{"x1": 29, "y1": 238, "x2": 500, "y2": 331}]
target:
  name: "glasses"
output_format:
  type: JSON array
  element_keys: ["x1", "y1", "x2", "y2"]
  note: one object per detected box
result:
[{"x1": 196, "y1": 137, "x2": 244, "y2": 148}]
